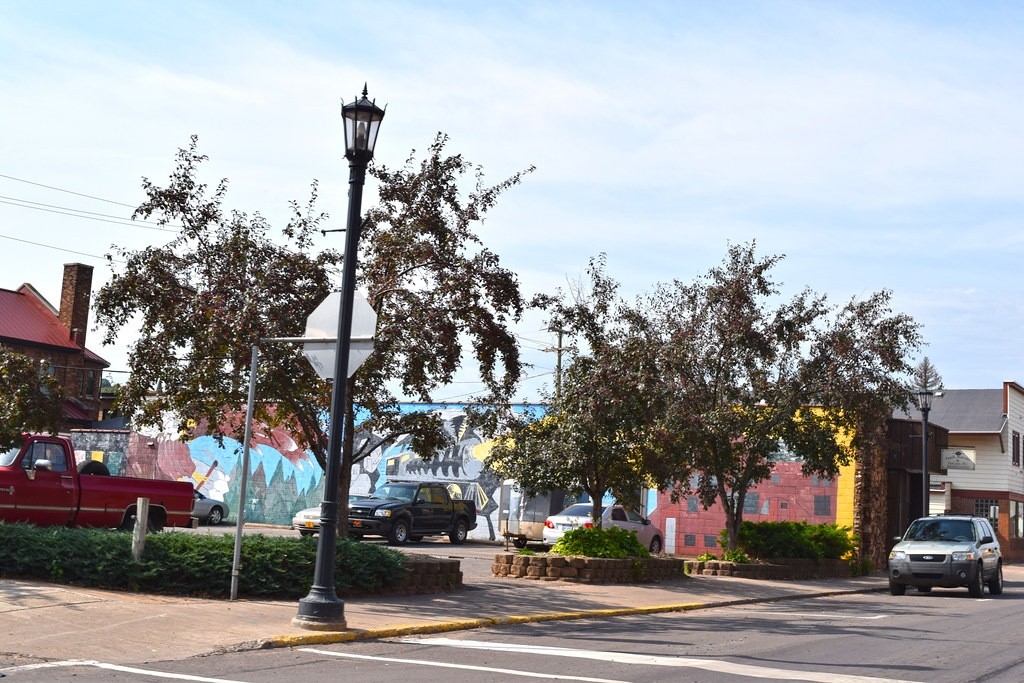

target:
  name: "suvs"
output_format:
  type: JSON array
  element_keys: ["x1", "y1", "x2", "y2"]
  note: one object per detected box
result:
[{"x1": 888, "y1": 515, "x2": 1003, "y2": 597}]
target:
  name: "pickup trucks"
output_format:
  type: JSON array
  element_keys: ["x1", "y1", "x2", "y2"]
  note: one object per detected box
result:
[
  {"x1": 0, "y1": 434, "x2": 194, "y2": 536},
  {"x1": 348, "y1": 480, "x2": 477, "y2": 545}
]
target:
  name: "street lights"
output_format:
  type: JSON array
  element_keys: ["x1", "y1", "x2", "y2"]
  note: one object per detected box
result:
[
  {"x1": 298, "y1": 82, "x2": 389, "y2": 621},
  {"x1": 915, "y1": 384, "x2": 934, "y2": 516}
]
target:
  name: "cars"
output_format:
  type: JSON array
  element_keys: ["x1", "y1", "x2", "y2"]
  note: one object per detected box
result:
[
  {"x1": 292, "y1": 494, "x2": 372, "y2": 540},
  {"x1": 542, "y1": 502, "x2": 663, "y2": 555},
  {"x1": 194, "y1": 487, "x2": 229, "y2": 525}
]
